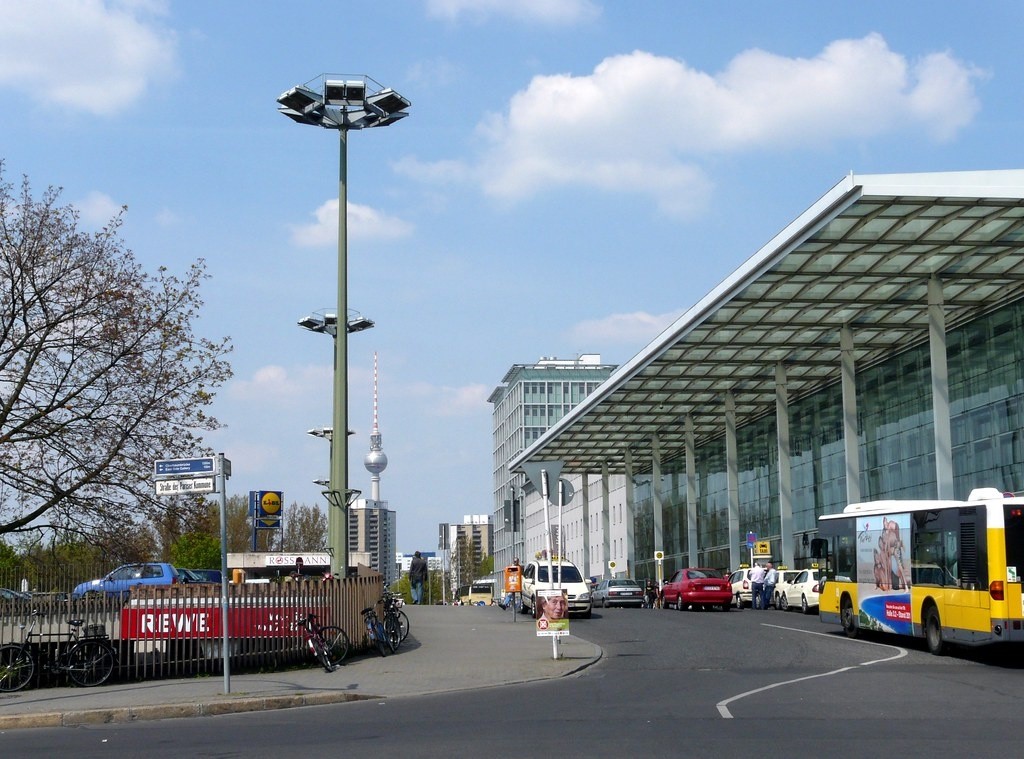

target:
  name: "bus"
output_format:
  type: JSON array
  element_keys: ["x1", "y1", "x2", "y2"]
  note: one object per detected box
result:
[
  {"x1": 454, "y1": 583, "x2": 494, "y2": 606},
  {"x1": 810, "y1": 488, "x2": 1023, "y2": 657}
]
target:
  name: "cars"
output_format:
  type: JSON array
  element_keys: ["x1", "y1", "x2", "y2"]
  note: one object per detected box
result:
[
  {"x1": 721, "y1": 564, "x2": 776, "y2": 610},
  {"x1": 72, "y1": 561, "x2": 180, "y2": 600},
  {"x1": 521, "y1": 557, "x2": 592, "y2": 620},
  {"x1": 177, "y1": 568, "x2": 223, "y2": 584},
  {"x1": 590, "y1": 578, "x2": 645, "y2": 608},
  {"x1": 660, "y1": 566, "x2": 733, "y2": 612},
  {"x1": 755, "y1": 562, "x2": 833, "y2": 615},
  {"x1": 0, "y1": 587, "x2": 35, "y2": 602}
]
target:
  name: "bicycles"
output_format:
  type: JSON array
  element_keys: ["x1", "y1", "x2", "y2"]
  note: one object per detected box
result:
[
  {"x1": 361, "y1": 591, "x2": 410, "y2": 658},
  {"x1": 0, "y1": 609, "x2": 118, "y2": 692},
  {"x1": 296, "y1": 613, "x2": 349, "y2": 672}
]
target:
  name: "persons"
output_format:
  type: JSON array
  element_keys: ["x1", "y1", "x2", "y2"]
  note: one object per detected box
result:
[
  {"x1": 749, "y1": 561, "x2": 765, "y2": 610},
  {"x1": 539, "y1": 593, "x2": 566, "y2": 620},
  {"x1": 498, "y1": 556, "x2": 524, "y2": 611},
  {"x1": 759, "y1": 561, "x2": 777, "y2": 610},
  {"x1": 645, "y1": 580, "x2": 657, "y2": 609},
  {"x1": 409, "y1": 551, "x2": 428, "y2": 605}
]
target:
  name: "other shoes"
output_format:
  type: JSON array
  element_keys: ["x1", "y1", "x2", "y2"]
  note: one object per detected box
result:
[
  {"x1": 413, "y1": 600, "x2": 418, "y2": 604},
  {"x1": 498, "y1": 604, "x2": 506, "y2": 610}
]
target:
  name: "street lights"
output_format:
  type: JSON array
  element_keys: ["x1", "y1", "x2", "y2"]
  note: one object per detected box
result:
[
  {"x1": 274, "y1": 70, "x2": 412, "y2": 577},
  {"x1": 291, "y1": 306, "x2": 376, "y2": 494}
]
